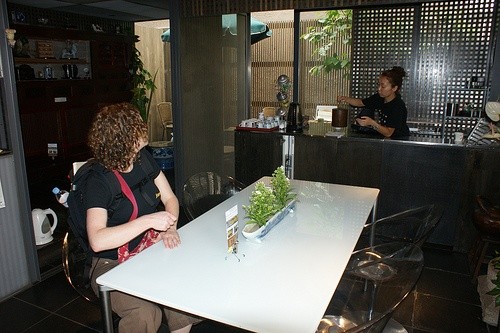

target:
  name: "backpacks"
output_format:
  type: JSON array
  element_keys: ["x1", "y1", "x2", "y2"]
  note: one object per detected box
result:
[{"x1": 67, "y1": 156, "x2": 152, "y2": 254}]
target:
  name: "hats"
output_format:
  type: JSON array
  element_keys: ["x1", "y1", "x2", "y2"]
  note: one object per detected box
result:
[{"x1": 485, "y1": 102, "x2": 500, "y2": 122}]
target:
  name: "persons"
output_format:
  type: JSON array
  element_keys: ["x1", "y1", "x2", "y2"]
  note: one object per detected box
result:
[
  {"x1": 67, "y1": 102, "x2": 206, "y2": 333},
  {"x1": 338, "y1": 66, "x2": 411, "y2": 141}
]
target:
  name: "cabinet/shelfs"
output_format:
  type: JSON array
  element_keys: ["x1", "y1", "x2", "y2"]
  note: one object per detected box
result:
[
  {"x1": 442, "y1": 85, "x2": 491, "y2": 140},
  {"x1": 11, "y1": 24, "x2": 127, "y2": 169}
]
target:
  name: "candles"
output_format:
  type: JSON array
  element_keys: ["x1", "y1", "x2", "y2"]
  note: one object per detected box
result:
[{"x1": 242, "y1": 167, "x2": 297, "y2": 239}]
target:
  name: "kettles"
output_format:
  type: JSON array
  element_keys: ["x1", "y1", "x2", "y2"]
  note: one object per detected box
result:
[
  {"x1": 286, "y1": 102, "x2": 303, "y2": 134},
  {"x1": 32, "y1": 208, "x2": 57, "y2": 246}
]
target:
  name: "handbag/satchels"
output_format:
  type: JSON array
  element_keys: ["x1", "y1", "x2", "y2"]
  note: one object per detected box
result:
[{"x1": 118, "y1": 229, "x2": 160, "y2": 265}]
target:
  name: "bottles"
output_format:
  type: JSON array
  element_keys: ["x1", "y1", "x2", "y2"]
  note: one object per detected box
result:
[{"x1": 52, "y1": 187, "x2": 69, "y2": 208}]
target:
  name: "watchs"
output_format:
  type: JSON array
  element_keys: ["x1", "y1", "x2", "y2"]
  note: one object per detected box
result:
[{"x1": 373, "y1": 123, "x2": 379, "y2": 130}]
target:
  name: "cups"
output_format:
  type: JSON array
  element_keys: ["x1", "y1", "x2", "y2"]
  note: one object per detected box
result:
[
  {"x1": 239, "y1": 119, "x2": 285, "y2": 129},
  {"x1": 331, "y1": 108, "x2": 348, "y2": 127},
  {"x1": 455, "y1": 132, "x2": 463, "y2": 141}
]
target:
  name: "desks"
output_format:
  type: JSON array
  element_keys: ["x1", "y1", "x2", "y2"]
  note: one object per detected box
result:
[{"x1": 97, "y1": 177, "x2": 380, "y2": 333}]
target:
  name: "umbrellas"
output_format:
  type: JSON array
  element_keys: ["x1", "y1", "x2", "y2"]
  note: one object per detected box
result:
[{"x1": 161, "y1": 14, "x2": 272, "y2": 48}]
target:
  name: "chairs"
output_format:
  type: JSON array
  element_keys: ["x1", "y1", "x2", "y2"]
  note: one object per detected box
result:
[
  {"x1": 315, "y1": 204, "x2": 447, "y2": 333},
  {"x1": 183, "y1": 172, "x2": 245, "y2": 221},
  {"x1": 62, "y1": 161, "x2": 170, "y2": 332},
  {"x1": 158, "y1": 102, "x2": 172, "y2": 140},
  {"x1": 470, "y1": 194, "x2": 500, "y2": 285}
]
